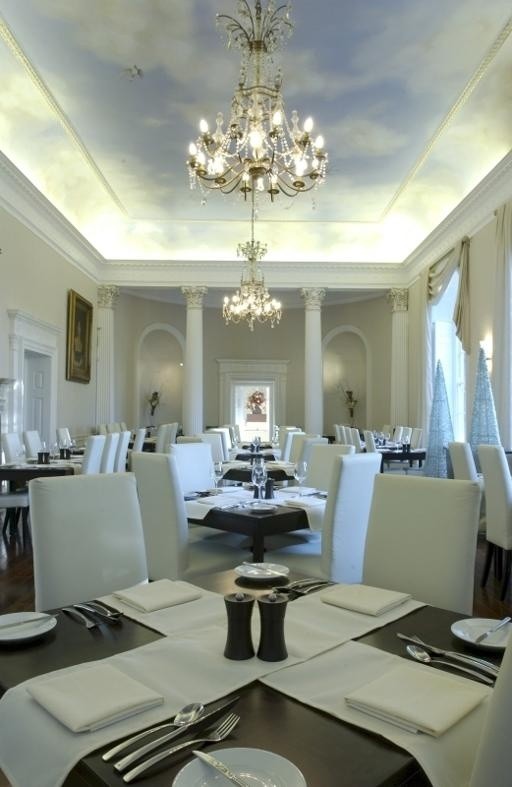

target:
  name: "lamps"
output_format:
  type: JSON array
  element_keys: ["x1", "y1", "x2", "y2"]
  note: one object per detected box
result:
[
  {"x1": 222, "y1": 177, "x2": 282, "y2": 332},
  {"x1": 185, "y1": 0, "x2": 329, "y2": 210}
]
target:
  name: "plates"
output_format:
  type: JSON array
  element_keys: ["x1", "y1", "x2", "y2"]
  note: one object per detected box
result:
[
  {"x1": 171, "y1": 747, "x2": 307, "y2": 787},
  {"x1": 234, "y1": 562, "x2": 290, "y2": 579},
  {"x1": 0, "y1": 612, "x2": 57, "y2": 641},
  {"x1": 450, "y1": 617, "x2": 512, "y2": 648},
  {"x1": 247, "y1": 503, "x2": 277, "y2": 513}
]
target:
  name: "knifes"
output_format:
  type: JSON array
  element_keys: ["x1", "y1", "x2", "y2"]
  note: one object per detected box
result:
[
  {"x1": 474, "y1": 617, "x2": 511, "y2": 644},
  {"x1": 191, "y1": 749, "x2": 249, "y2": 787},
  {"x1": 243, "y1": 561, "x2": 288, "y2": 577},
  {"x1": 72, "y1": 603, "x2": 118, "y2": 621},
  {"x1": 397, "y1": 632, "x2": 500, "y2": 672},
  {"x1": 112, "y1": 695, "x2": 241, "y2": 772},
  {"x1": 0, "y1": 613, "x2": 59, "y2": 629}
]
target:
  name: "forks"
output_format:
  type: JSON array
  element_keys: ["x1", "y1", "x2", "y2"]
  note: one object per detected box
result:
[
  {"x1": 122, "y1": 712, "x2": 240, "y2": 783},
  {"x1": 61, "y1": 607, "x2": 103, "y2": 629}
]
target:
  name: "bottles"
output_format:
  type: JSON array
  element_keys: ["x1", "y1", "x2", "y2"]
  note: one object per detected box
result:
[
  {"x1": 223, "y1": 593, "x2": 255, "y2": 660},
  {"x1": 255, "y1": 593, "x2": 289, "y2": 662}
]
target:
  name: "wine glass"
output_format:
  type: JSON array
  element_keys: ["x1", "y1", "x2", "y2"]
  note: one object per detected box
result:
[
  {"x1": 16, "y1": 433, "x2": 77, "y2": 468},
  {"x1": 293, "y1": 460, "x2": 311, "y2": 500},
  {"x1": 125, "y1": 423, "x2": 158, "y2": 442},
  {"x1": 370, "y1": 429, "x2": 410, "y2": 452},
  {"x1": 251, "y1": 465, "x2": 267, "y2": 499},
  {"x1": 208, "y1": 461, "x2": 224, "y2": 496},
  {"x1": 231, "y1": 430, "x2": 280, "y2": 453}
]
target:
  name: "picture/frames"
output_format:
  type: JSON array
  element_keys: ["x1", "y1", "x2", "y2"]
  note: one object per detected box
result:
[{"x1": 66, "y1": 288, "x2": 93, "y2": 384}]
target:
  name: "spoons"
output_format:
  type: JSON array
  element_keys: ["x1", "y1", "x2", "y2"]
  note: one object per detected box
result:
[
  {"x1": 274, "y1": 577, "x2": 333, "y2": 594},
  {"x1": 406, "y1": 644, "x2": 493, "y2": 684},
  {"x1": 84, "y1": 601, "x2": 123, "y2": 618},
  {"x1": 102, "y1": 702, "x2": 205, "y2": 762},
  {"x1": 412, "y1": 634, "x2": 499, "y2": 676}
]
